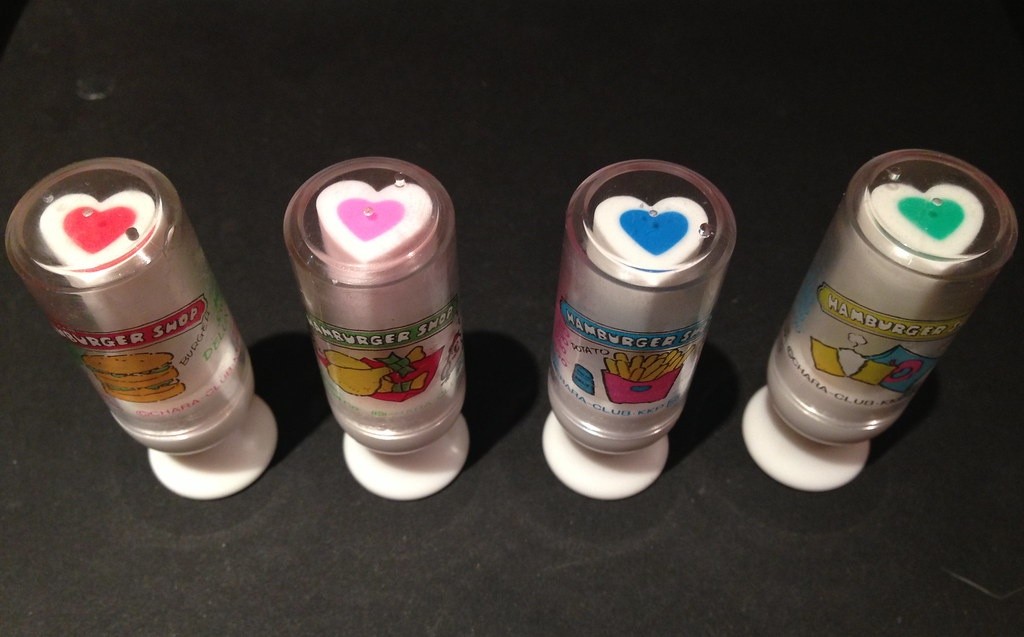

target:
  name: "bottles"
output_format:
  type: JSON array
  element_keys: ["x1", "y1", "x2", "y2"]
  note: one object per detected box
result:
[
  {"x1": 5, "y1": 157, "x2": 278, "y2": 501},
  {"x1": 542, "y1": 160, "x2": 737, "y2": 498},
  {"x1": 744, "y1": 148, "x2": 1019, "y2": 491},
  {"x1": 285, "y1": 158, "x2": 469, "y2": 502}
]
